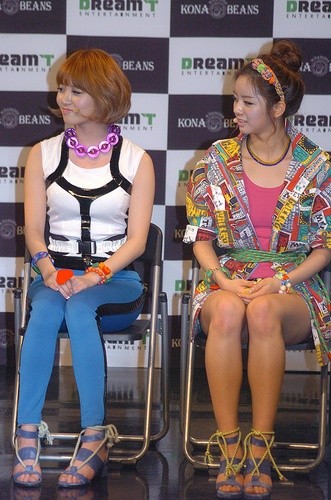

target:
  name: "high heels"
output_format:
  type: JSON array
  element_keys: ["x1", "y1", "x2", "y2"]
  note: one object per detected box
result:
[
  {"x1": 10, "y1": 427, "x2": 44, "y2": 487},
  {"x1": 244, "y1": 435, "x2": 275, "y2": 499},
  {"x1": 216, "y1": 433, "x2": 244, "y2": 500},
  {"x1": 56, "y1": 430, "x2": 112, "y2": 488}
]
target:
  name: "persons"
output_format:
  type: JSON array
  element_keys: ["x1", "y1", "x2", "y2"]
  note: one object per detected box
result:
[
  {"x1": 11, "y1": 49, "x2": 155, "y2": 488},
  {"x1": 181, "y1": 41, "x2": 331, "y2": 500}
]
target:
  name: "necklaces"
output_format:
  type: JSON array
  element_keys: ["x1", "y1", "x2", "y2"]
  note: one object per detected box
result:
[
  {"x1": 246, "y1": 135, "x2": 291, "y2": 166},
  {"x1": 64, "y1": 123, "x2": 121, "y2": 159}
]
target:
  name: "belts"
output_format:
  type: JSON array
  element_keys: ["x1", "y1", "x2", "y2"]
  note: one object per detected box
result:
[{"x1": 47, "y1": 235, "x2": 127, "y2": 256}]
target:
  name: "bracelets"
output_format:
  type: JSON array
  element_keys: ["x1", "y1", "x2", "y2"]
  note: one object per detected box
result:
[
  {"x1": 85, "y1": 262, "x2": 113, "y2": 286},
  {"x1": 202, "y1": 267, "x2": 231, "y2": 290},
  {"x1": 273, "y1": 271, "x2": 291, "y2": 295},
  {"x1": 30, "y1": 252, "x2": 54, "y2": 276}
]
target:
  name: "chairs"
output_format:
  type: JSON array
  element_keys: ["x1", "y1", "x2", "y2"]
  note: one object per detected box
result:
[
  {"x1": 12, "y1": 225, "x2": 168, "y2": 469},
  {"x1": 179, "y1": 242, "x2": 331, "y2": 480}
]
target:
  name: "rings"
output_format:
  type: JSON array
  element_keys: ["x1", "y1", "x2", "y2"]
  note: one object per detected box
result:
[{"x1": 55, "y1": 269, "x2": 74, "y2": 285}]
